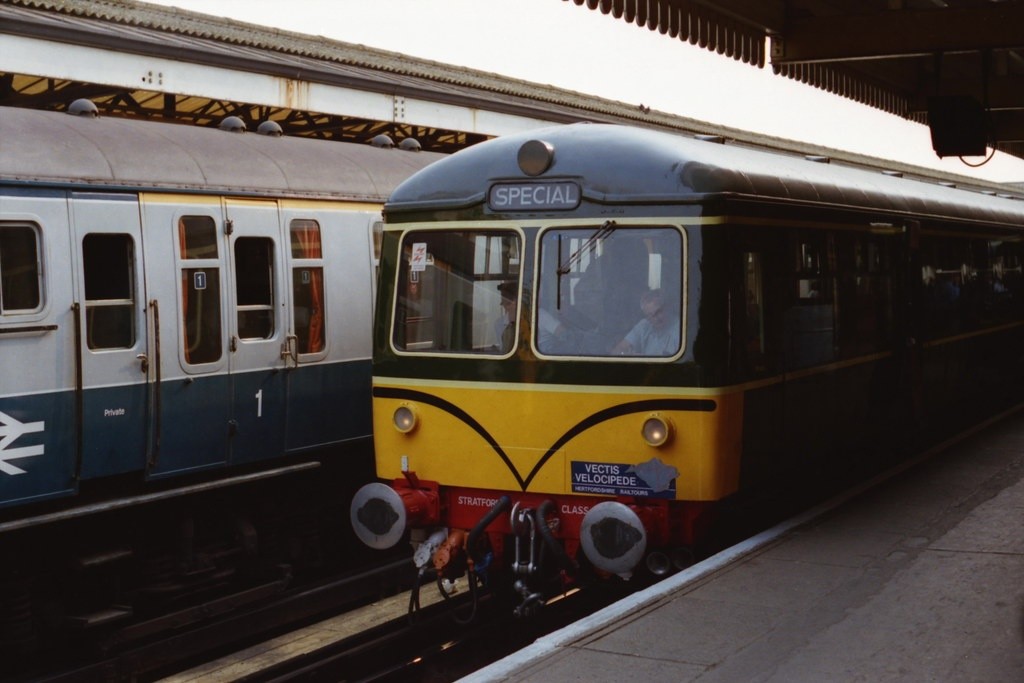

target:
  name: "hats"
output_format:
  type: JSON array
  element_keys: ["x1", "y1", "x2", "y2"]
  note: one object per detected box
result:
[{"x1": 497, "y1": 281, "x2": 532, "y2": 306}]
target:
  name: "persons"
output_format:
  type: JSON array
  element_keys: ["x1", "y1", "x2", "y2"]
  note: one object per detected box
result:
[
  {"x1": 611, "y1": 288, "x2": 680, "y2": 357},
  {"x1": 574, "y1": 240, "x2": 651, "y2": 326},
  {"x1": 491, "y1": 283, "x2": 574, "y2": 355}
]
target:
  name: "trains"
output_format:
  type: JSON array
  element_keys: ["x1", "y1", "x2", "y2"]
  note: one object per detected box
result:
[
  {"x1": 348, "y1": 121, "x2": 1024, "y2": 624},
  {"x1": 0, "y1": 97, "x2": 454, "y2": 540}
]
